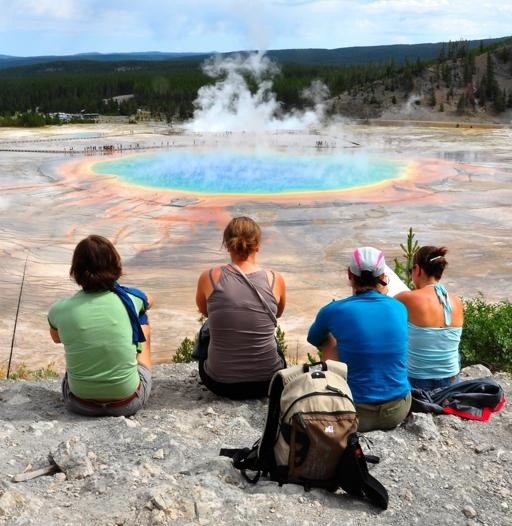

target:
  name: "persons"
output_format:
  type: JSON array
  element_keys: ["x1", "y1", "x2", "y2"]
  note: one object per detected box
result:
[
  {"x1": 196, "y1": 216, "x2": 287, "y2": 401},
  {"x1": 393, "y1": 246, "x2": 464, "y2": 392},
  {"x1": 47, "y1": 235, "x2": 154, "y2": 417},
  {"x1": 307, "y1": 246, "x2": 412, "y2": 433}
]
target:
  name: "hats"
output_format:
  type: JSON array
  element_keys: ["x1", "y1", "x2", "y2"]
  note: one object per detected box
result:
[{"x1": 349, "y1": 246, "x2": 386, "y2": 280}]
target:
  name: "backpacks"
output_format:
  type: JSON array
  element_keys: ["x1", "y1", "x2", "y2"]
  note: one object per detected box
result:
[{"x1": 255, "y1": 359, "x2": 360, "y2": 491}]
